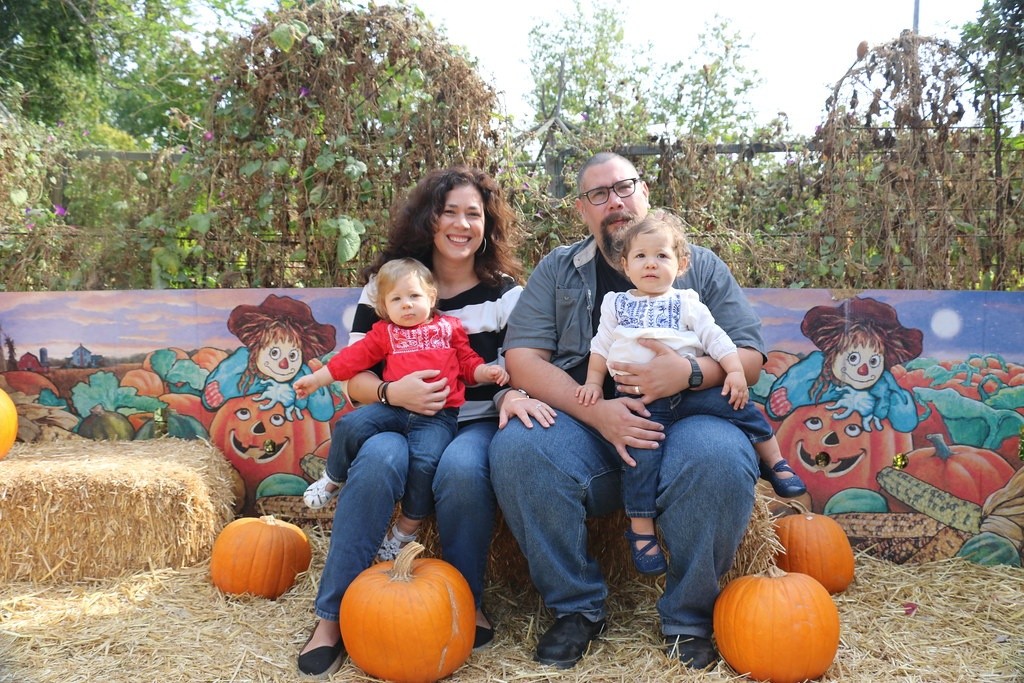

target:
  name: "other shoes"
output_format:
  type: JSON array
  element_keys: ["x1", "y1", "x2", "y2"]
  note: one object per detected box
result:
[
  {"x1": 473, "y1": 620, "x2": 494, "y2": 654},
  {"x1": 666, "y1": 634, "x2": 715, "y2": 673},
  {"x1": 759, "y1": 458, "x2": 807, "y2": 497},
  {"x1": 534, "y1": 616, "x2": 607, "y2": 670},
  {"x1": 297, "y1": 619, "x2": 343, "y2": 680},
  {"x1": 625, "y1": 529, "x2": 668, "y2": 576}
]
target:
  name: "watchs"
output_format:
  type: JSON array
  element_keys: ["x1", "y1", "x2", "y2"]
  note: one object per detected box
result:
[{"x1": 686, "y1": 357, "x2": 703, "y2": 389}]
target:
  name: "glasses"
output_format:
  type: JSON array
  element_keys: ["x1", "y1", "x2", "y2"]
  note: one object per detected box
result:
[{"x1": 579, "y1": 178, "x2": 641, "y2": 206}]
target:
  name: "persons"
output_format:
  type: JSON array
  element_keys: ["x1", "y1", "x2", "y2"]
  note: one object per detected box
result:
[
  {"x1": 293, "y1": 257, "x2": 511, "y2": 566},
  {"x1": 573, "y1": 207, "x2": 808, "y2": 575},
  {"x1": 296, "y1": 167, "x2": 557, "y2": 682},
  {"x1": 488, "y1": 152, "x2": 769, "y2": 672}
]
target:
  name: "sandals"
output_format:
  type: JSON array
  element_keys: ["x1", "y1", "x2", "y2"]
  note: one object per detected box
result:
[
  {"x1": 378, "y1": 525, "x2": 417, "y2": 561},
  {"x1": 303, "y1": 473, "x2": 346, "y2": 509}
]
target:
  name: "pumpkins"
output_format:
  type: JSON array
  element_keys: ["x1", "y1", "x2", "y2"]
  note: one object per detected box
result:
[
  {"x1": 0, "y1": 388, "x2": 18, "y2": 460},
  {"x1": 339, "y1": 541, "x2": 476, "y2": 683},
  {"x1": 771, "y1": 500, "x2": 855, "y2": 595},
  {"x1": 210, "y1": 515, "x2": 312, "y2": 601},
  {"x1": 713, "y1": 565, "x2": 839, "y2": 683}
]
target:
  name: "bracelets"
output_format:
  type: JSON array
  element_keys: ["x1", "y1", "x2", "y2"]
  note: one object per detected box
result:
[
  {"x1": 377, "y1": 380, "x2": 395, "y2": 406},
  {"x1": 518, "y1": 389, "x2": 529, "y2": 399}
]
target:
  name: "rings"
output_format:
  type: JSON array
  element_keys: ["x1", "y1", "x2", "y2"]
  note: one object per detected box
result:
[
  {"x1": 635, "y1": 386, "x2": 641, "y2": 394},
  {"x1": 536, "y1": 404, "x2": 542, "y2": 409}
]
